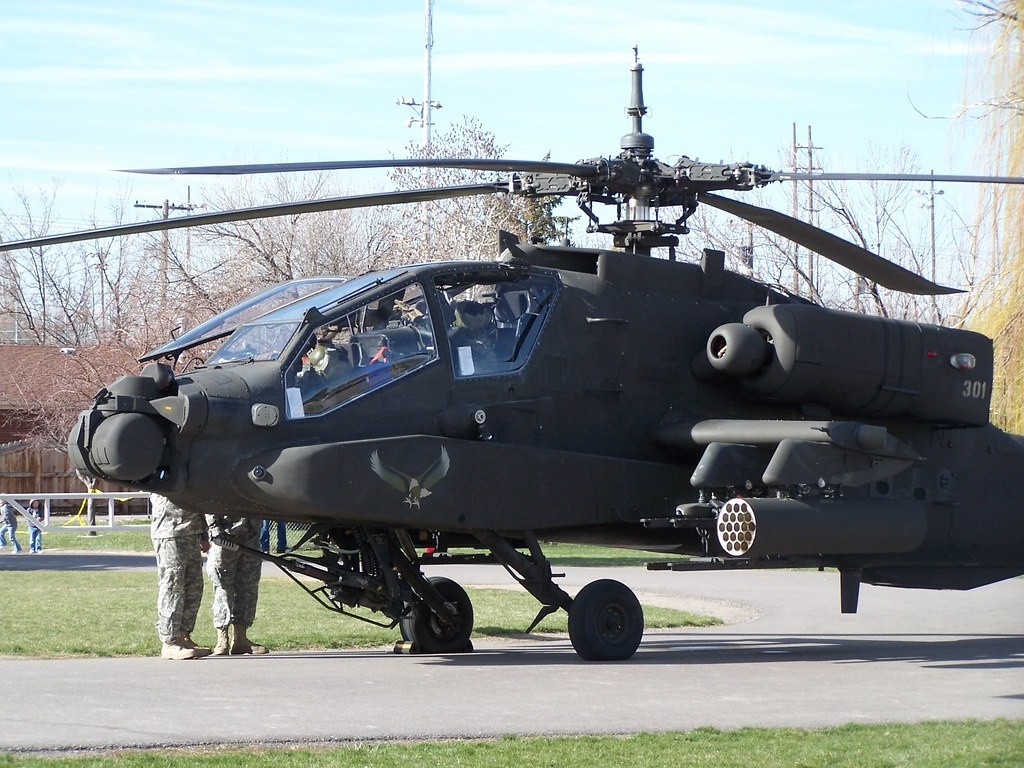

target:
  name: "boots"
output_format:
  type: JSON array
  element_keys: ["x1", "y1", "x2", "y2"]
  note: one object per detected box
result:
[{"x1": 214, "y1": 624, "x2": 269, "y2": 654}]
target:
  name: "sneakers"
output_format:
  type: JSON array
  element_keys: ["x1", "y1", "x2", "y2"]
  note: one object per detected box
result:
[{"x1": 161, "y1": 637, "x2": 212, "y2": 661}]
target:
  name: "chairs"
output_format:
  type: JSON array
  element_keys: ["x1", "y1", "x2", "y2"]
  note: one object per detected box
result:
[{"x1": 490, "y1": 291, "x2": 528, "y2": 361}]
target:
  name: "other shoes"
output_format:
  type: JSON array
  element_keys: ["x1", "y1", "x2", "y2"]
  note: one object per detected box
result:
[
  {"x1": 11, "y1": 550, "x2": 21, "y2": 555},
  {"x1": 30, "y1": 550, "x2": 34, "y2": 554},
  {"x1": 37, "y1": 551, "x2": 44, "y2": 555},
  {"x1": 0, "y1": 545, "x2": 8, "y2": 549}
]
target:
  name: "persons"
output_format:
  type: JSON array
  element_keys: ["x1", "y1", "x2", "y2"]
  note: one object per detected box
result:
[
  {"x1": 25, "y1": 499, "x2": 44, "y2": 555},
  {"x1": 206, "y1": 514, "x2": 270, "y2": 655},
  {"x1": 261, "y1": 519, "x2": 291, "y2": 554},
  {"x1": 150, "y1": 491, "x2": 213, "y2": 661},
  {"x1": 0, "y1": 493, "x2": 22, "y2": 554},
  {"x1": 391, "y1": 298, "x2": 496, "y2": 373}
]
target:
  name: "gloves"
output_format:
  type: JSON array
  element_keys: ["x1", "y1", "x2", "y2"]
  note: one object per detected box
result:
[{"x1": 392, "y1": 299, "x2": 429, "y2": 323}]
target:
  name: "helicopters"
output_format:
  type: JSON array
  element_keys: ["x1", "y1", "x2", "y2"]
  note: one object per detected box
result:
[{"x1": 1, "y1": 44, "x2": 1024, "y2": 663}]
